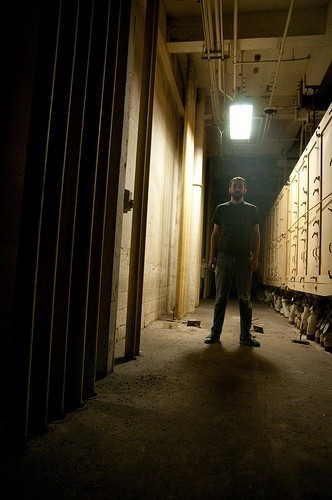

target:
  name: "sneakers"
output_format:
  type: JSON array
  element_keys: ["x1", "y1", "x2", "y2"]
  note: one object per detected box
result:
[{"x1": 204, "y1": 332, "x2": 220, "y2": 344}]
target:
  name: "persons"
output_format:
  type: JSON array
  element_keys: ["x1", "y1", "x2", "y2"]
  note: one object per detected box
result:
[{"x1": 205, "y1": 176, "x2": 260, "y2": 348}]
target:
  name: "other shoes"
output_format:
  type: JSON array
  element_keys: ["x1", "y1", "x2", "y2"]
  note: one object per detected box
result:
[{"x1": 239, "y1": 334, "x2": 261, "y2": 347}]
